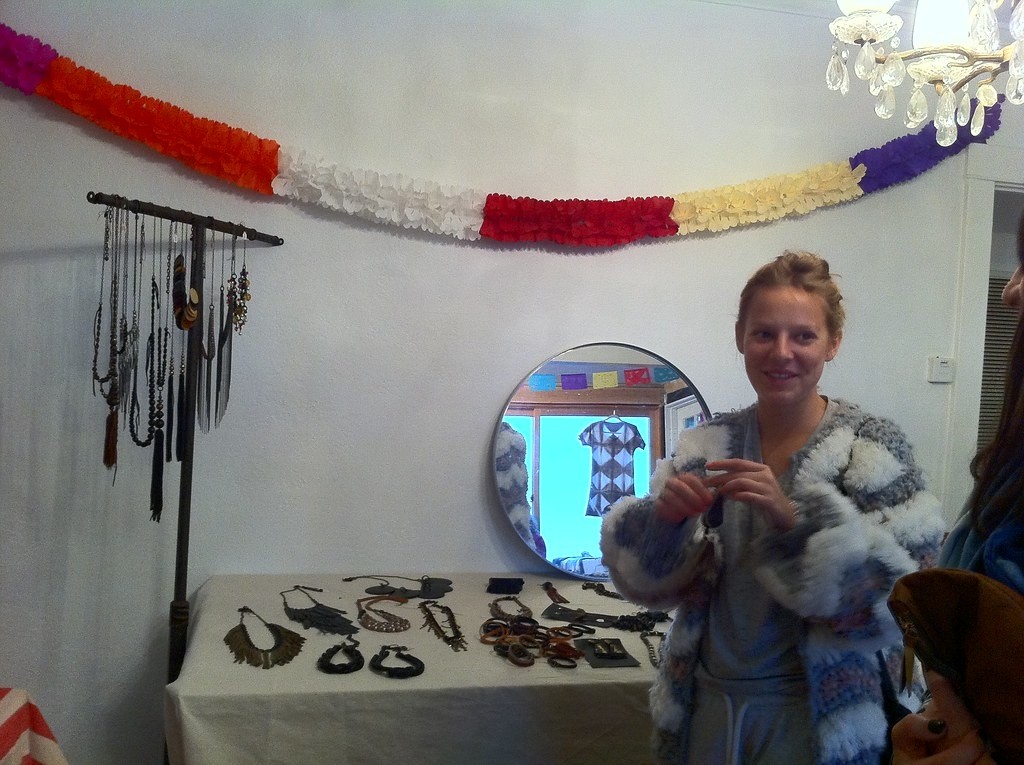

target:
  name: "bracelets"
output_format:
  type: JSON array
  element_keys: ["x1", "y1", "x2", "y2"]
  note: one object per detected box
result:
[
  {"x1": 479, "y1": 616, "x2": 595, "y2": 669},
  {"x1": 789, "y1": 500, "x2": 801, "y2": 522}
]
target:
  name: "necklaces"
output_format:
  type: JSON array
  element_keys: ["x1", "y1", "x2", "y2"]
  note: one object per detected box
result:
[
  {"x1": 280, "y1": 585, "x2": 360, "y2": 635},
  {"x1": 356, "y1": 595, "x2": 410, "y2": 633},
  {"x1": 641, "y1": 631, "x2": 666, "y2": 667},
  {"x1": 418, "y1": 600, "x2": 467, "y2": 653},
  {"x1": 343, "y1": 574, "x2": 454, "y2": 599},
  {"x1": 223, "y1": 606, "x2": 305, "y2": 668},
  {"x1": 91, "y1": 194, "x2": 249, "y2": 521}
]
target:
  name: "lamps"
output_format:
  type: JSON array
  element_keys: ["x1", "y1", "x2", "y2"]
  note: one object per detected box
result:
[{"x1": 822, "y1": 0, "x2": 1024, "y2": 149}]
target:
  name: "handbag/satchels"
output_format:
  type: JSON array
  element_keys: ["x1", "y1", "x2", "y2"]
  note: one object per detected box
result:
[{"x1": 885, "y1": 569, "x2": 1024, "y2": 765}]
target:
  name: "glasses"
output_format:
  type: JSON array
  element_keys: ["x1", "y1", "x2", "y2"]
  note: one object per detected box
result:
[{"x1": 693, "y1": 488, "x2": 726, "y2": 573}]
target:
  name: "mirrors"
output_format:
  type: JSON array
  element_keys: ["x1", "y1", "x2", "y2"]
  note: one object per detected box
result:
[{"x1": 490, "y1": 341, "x2": 721, "y2": 579}]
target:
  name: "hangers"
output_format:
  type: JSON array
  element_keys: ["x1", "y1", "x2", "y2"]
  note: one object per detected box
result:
[{"x1": 600, "y1": 404, "x2": 625, "y2": 424}]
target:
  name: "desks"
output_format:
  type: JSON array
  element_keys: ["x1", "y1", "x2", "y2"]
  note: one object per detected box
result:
[{"x1": 162, "y1": 565, "x2": 675, "y2": 765}]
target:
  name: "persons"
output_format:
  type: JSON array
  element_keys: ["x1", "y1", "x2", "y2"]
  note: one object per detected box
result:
[
  {"x1": 889, "y1": 200, "x2": 1024, "y2": 765},
  {"x1": 604, "y1": 253, "x2": 943, "y2": 765},
  {"x1": 496, "y1": 421, "x2": 536, "y2": 548}
]
